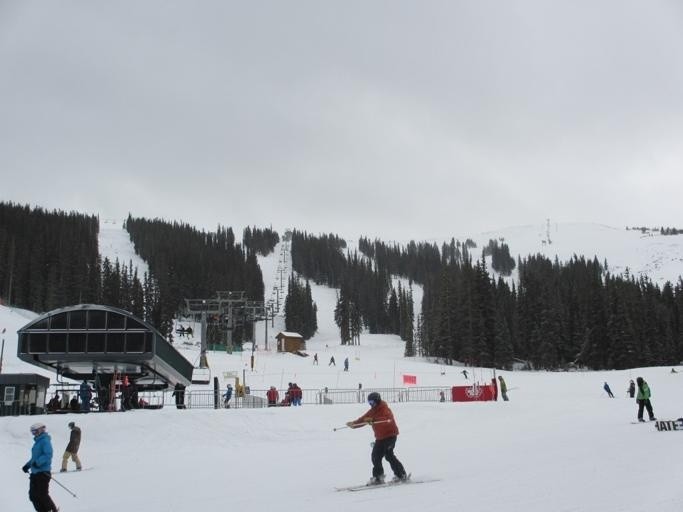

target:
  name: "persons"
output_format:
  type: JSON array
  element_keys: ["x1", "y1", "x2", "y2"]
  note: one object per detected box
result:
[
  {"x1": 222, "y1": 384, "x2": 233, "y2": 408},
  {"x1": 48, "y1": 379, "x2": 186, "y2": 414},
  {"x1": 313, "y1": 353, "x2": 318, "y2": 365},
  {"x1": 21, "y1": 422, "x2": 57, "y2": 512},
  {"x1": 329, "y1": 356, "x2": 335, "y2": 365},
  {"x1": 346, "y1": 393, "x2": 405, "y2": 487},
  {"x1": 636, "y1": 376, "x2": 657, "y2": 422},
  {"x1": 460, "y1": 369, "x2": 468, "y2": 378},
  {"x1": 266, "y1": 381, "x2": 302, "y2": 407},
  {"x1": 604, "y1": 382, "x2": 615, "y2": 398},
  {"x1": 343, "y1": 358, "x2": 349, "y2": 371},
  {"x1": 498, "y1": 376, "x2": 509, "y2": 401},
  {"x1": 59, "y1": 421, "x2": 83, "y2": 472},
  {"x1": 626, "y1": 380, "x2": 635, "y2": 398}
]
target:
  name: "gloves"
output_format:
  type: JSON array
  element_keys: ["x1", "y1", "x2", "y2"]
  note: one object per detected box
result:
[
  {"x1": 23, "y1": 465, "x2": 29, "y2": 473},
  {"x1": 32, "y1": 461, "x2": 40, "y2": 469}
]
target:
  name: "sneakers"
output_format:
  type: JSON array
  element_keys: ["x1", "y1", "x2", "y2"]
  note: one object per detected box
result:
[
  {"x1": 638, "y1": 417, "x2": 645, "y2": 422},
  {"x1": 60, "y1": 468, "x2": 67, "y2": 472},
  {"x1": 649, "y1": 417, "x2": 657, "y2": 421},
  {"x1": 366, "y1": 475, "x2": 385, "y2": 486},
  {"x1": 76, "y1": 466, "x2": 82, "y2": 470},
  {"x1": 392, "y1": 472, "x2": 407, "y2": 483}
]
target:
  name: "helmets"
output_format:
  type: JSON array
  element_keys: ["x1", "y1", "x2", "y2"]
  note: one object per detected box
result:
[
  {"x1": 68, "y1": 422, "x2": 75, "y2": 428},
  {"x1": 30, "y1": 422, "x2": 46, "y2": 431},
  {"x1": 367, "y1": 392, "x2": 381, "y2": 407},
  {"x1": 637, "y1": 377, "x2": 643, "y2": 383}
]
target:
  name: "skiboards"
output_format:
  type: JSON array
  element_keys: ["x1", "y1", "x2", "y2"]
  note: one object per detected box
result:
[
  {"x1": 51, "y1": 468, "x2": 93, "y2": 474},
  {"x1": 335, "y1": 479, "x2": 441, "y2": 492}
]
